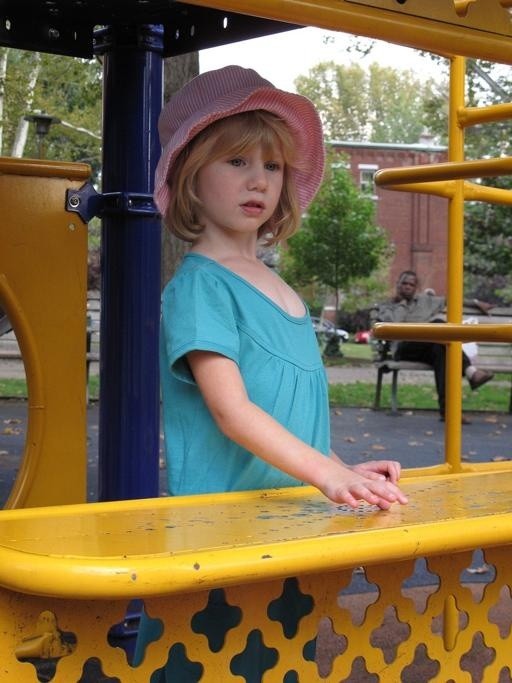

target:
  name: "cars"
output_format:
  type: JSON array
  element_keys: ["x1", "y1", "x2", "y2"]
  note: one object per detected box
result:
[{"x1": 310, "y1": 316, "x2": 373, "y2": 344}]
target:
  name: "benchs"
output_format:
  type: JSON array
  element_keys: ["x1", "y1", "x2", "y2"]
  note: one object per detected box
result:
[{"x1": 368, "y1": 311, "x2": 511, "y2": 422}]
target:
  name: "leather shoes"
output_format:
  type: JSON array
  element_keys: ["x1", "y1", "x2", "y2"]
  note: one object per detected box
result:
[
  {"x1": 439, "y1": 415, "x2": 471, "y2": 424},
  {"x1": 467, "y1": 370, "x2": 494, "y2": 390}
]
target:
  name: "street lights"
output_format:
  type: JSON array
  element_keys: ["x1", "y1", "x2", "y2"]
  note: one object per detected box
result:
[{"x1": 24, "y1": 109, "x2": 61, "y2": 159}]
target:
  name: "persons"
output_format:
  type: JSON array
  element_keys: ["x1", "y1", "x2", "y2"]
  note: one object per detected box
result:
[
  {"x1": 132, "y1": 66, "x2": 408, "y2": 682},
  {"x1": 379, "y1": 270, "x2": 497, "y2": 425}
]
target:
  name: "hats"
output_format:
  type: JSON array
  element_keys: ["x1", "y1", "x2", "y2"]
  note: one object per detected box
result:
[{"x1": 153, "y1": 65, "x2": 325, "y2": 237}]
